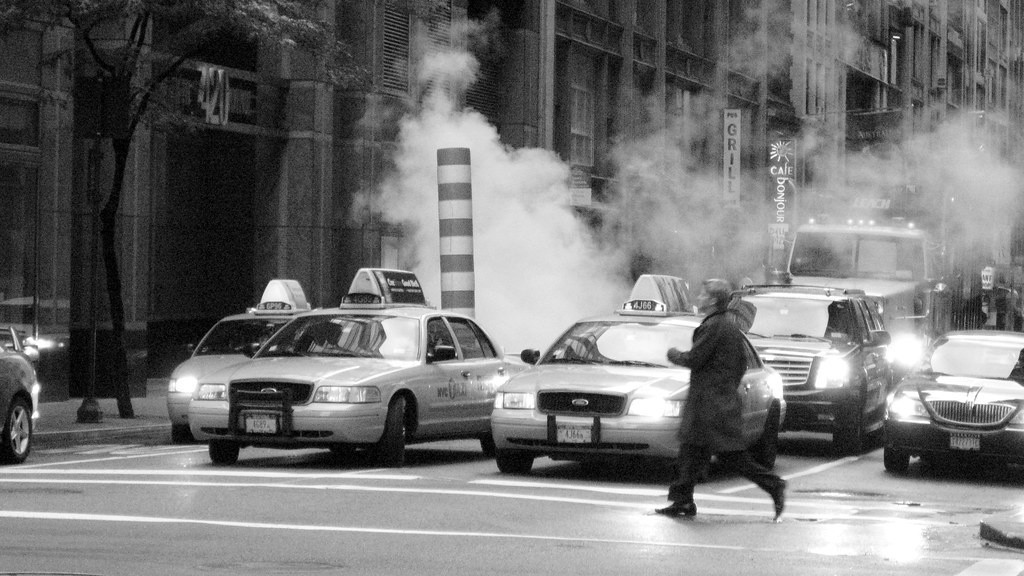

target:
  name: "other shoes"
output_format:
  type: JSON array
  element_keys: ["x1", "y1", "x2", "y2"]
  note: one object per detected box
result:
[
  {"x1": 771, "y1": 478, "x2": 786, "y2": 518},
  {"x1": 656, "y1": 503, "x2": 697, "y2": 518}
]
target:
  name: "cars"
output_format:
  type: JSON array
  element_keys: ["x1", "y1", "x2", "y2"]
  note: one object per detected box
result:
[
  {"x1": 489, "y1": 273, "x2": 786, "y2": 485},
  {"x1": 0, "y1": 339, "x2": 40, "y2": 467},
  {"x1": 881, "y1": 328, "x2": 1024, "y2": 487},
  {"x1": 188, "y1": 266, "x2": 531, "y2": 467},
  {"x1": 167, "y1": 279, "x2": 324, "y2": 447}
]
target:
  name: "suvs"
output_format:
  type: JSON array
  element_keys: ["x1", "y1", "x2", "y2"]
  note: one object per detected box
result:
[{"x1": 731, "y1": 284, "x2": 894, "y2": 456}]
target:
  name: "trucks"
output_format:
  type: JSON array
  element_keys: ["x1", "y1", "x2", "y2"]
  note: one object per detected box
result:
[{"x1": 778, "y1": 211, "x2": 953, "y2": 351}]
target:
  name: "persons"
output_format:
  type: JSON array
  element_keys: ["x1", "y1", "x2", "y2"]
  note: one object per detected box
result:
[{"x1": 653, "y1": 277, "x2": 789, "y2": 521}]
